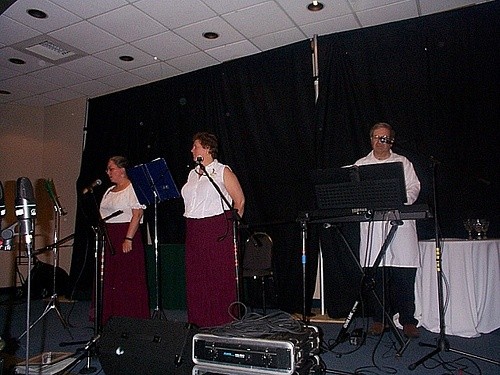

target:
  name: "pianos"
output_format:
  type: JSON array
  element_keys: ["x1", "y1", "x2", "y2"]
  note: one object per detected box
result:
[{"x1": 300, "y1": 202, "x2": 435, "y2": 359}]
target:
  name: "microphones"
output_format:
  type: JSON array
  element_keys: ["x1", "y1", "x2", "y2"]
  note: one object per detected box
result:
[
  {"x1": 195, "y1": 155, "x2": 204, "y2": 163},
  {"x1": 83, "y1": 179, "x2": 102, "y2": 194}
]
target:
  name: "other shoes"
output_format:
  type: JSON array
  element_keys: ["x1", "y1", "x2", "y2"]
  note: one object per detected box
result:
[
  {"x1": 369, "y1": 321, "x2": 390, "y2": 335},
  {"x1": 403, "y1": 324, "x2": 421, "y2": 338}
]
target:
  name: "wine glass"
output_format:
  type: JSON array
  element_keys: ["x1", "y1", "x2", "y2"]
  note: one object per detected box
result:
[
  {"x1": 472, "y1": 217, "x2": 483, "y2": 240},
  {"x1": 463, "y1": 218, "x2": 476, "y2": 241},
  {"x1": 480, "y1": 217, "x2": 490, "y2": 240}
]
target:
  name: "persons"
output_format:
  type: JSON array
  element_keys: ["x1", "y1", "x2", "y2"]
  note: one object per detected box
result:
[
  {"x1": 89, "y1": 157, "x2": 149, "y2": 328},
  {"x1": 181, "y1": 132, "x2": 245, "y2": 328},
  {"x1": 353, "y1": 122, "x2": 420, "y2": 339}
]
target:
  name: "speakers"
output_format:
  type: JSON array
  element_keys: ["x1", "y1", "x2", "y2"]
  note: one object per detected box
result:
[{"x1": 97, "y1": 315, "x2": 198, "y2": 375}]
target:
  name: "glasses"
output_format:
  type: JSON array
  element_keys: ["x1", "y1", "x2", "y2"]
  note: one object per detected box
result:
[
  {"x1": 105, "y1": 168, "x2": 117, "y2": 173},
  {"x1": 375, "y1": 135, "x2": 387, "y2": 139}
]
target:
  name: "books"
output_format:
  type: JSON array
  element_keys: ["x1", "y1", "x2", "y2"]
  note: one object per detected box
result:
[{"x1": 14, "y1": 352, "x2": 77, "y2": 375}]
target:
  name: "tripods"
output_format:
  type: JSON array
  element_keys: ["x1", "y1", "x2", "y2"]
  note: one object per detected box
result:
[
  {"x1": 15, "y1": 211, "x2": 74, "y2": 342},
  {"x1": 392, "y1": 142, "x2": 500, "y2": 369},
  {"x1": 58, "y1": 158, "x2": 179, "y2": 375}
]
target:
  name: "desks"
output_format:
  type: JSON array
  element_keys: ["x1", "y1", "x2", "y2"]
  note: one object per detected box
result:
[
  {"x1": 414, "y1": 238, "x2": 500, "y2": 338},
  {"x1": 145, "y1": 244, "x2": 257, "y2": 310}
]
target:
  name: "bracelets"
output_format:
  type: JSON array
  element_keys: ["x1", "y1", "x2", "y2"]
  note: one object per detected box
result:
[{"x1": 125, "y1": 237, "x2": 132, "y2": 240}]
